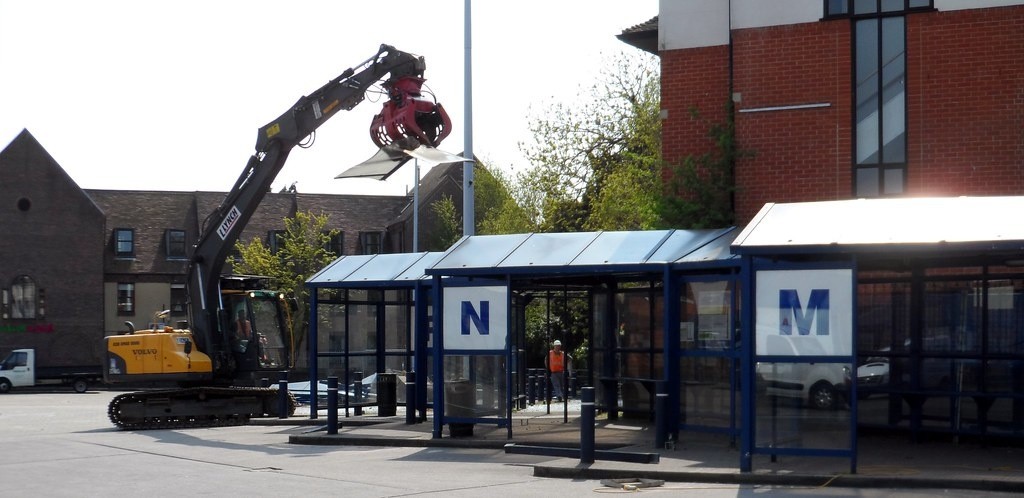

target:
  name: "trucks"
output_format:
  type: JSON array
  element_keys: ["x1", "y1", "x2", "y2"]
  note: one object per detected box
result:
[{"x1": 0, "y1": 349, "x2": 103, "y2": 394}]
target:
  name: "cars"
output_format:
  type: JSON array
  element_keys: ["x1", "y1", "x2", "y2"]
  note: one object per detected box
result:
[
  {"x1": 755, "y1": 326, "x2": 846, "y2": 415},
  {"x1": 856, "y1": 341, "x2": 912, "y2": 400}
]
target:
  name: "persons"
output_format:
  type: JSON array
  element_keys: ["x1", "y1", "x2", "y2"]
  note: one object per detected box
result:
[
  {"x1": 233, "y1": 310, "x2": 271, "y2": 364},
  {"x1": 545, "y1": 340, "x2": 572, "y2": 402}
]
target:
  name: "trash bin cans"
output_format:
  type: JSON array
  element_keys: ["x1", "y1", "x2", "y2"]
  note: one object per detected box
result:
[
  {"x1": 376, "y1": 373, "x2": 396, "y2": 417},
  {"x1": 445, "y1": 380, "x2": 475, "y2": 438}
]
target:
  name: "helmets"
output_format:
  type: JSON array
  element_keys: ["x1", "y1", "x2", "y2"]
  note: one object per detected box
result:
[{"x1": 554, "y1": 340, "x2": 561, "y2": 346}]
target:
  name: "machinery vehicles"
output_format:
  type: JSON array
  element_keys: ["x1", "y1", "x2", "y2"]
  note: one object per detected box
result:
[{"x1": 99, "y1": 43, "x2": 453, "y2": 432}]
